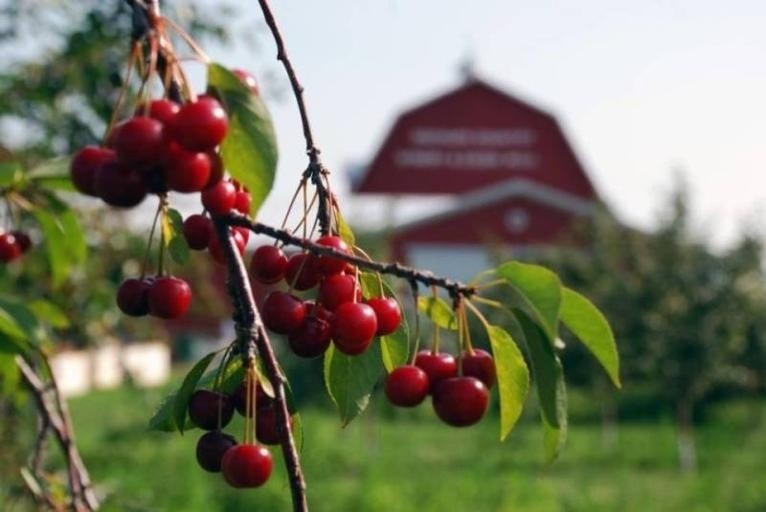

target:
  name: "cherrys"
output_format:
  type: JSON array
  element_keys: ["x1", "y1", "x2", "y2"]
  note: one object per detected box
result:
[{"x1": 0, "y1": 17, "x2": 496, "y2": 488}]
